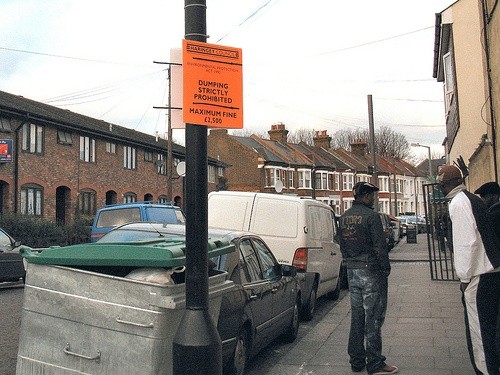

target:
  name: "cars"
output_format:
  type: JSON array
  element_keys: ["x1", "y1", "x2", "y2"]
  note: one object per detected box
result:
[
  {"x1": 94, "y1": 221, "x2": 302, "y2": 375},
  {"x1": 0, "y1": 227, "x2": 32, "y2": 285},
  {"x1": 389, "y1": 213, "x2": 431, "y2": 244}
]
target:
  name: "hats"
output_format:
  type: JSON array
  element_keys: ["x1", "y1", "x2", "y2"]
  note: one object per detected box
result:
[
  {"x1": 438, "y1": 166, "x2": 463, "y2": 184},
  {"x1": 352, "y1": 182, "x2": 379, "y2": 196},
  {"x1": 474, "y1": 182, "x2": 500, "y2": 195}
]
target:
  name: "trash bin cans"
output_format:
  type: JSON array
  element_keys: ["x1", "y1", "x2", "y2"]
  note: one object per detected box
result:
[
  {"x1": 406, "y1": 225, "x2": 417, "y2": 243},
  {"x1": 15, "y1": 239, "x2": 236, "y2": 375}
]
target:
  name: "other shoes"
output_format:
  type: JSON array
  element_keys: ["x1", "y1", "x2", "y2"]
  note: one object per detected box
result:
[{"x1": 371, "y1": 364, "x2": 398, "y2": 375}]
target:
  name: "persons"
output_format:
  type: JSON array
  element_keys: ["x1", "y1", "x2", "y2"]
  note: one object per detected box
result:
[
  {"x1": 474, "y1": 182, "x2": 500, "y2": 240},
  {"x1": 338, "y1": 181, "x2": 398, "y2": 375},
  {"x1": 439, "y1": 164, "x2": 500, "y2": 375}
]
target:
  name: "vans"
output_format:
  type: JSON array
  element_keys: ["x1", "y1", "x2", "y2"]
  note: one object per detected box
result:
[
  {"x1": 378, "y1": 212, "x2": 396, "y2": 252},
  {"x1": 208, "y1": 191, "x2": 342, "y2": 321},
  {"x1": 90, "y1": 201, "x2": 187, "y2": 243}
]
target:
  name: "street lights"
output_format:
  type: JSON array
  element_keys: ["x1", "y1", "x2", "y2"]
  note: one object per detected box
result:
[{"x1": 411, "y1": 143, "x2": 433, "y2": 183}]
